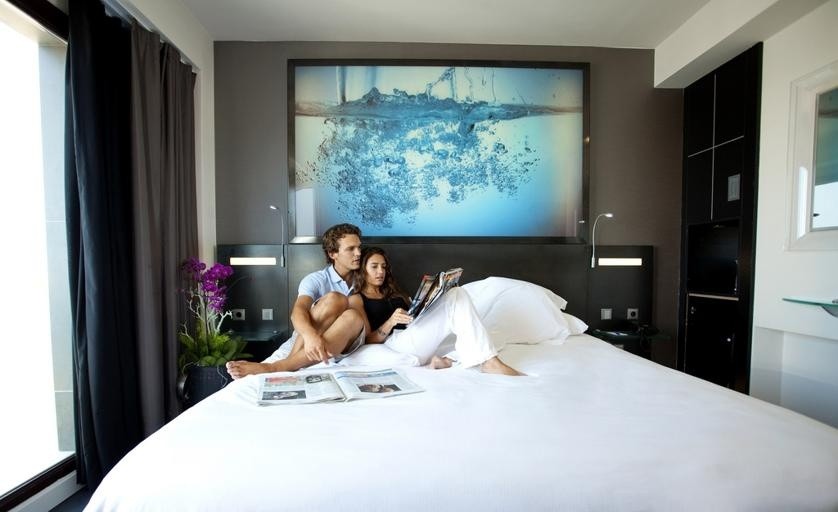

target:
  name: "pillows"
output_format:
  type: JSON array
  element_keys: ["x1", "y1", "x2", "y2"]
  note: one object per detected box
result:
[
  {"x1": 563, "y1": 313, "x2": 589, "y2": 341},
  {"x1": 538, "y1": 284, "x2": 569, "y2": 311},
  {"x1": 461, "y1": 275, "x2": 567, "y2": 346}
]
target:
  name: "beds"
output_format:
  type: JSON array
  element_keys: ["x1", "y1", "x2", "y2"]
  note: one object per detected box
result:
[{"x1": 84, "y1": 235, "x2": 836, "y2": 512}]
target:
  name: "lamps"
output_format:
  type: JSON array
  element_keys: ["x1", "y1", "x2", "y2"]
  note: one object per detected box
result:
[
  {"x1": 269, "y1": 204, "x2": 285, "y2": 268},
  {"x1": 590, "y1": 212, "x2": 615, "y2": 269}
]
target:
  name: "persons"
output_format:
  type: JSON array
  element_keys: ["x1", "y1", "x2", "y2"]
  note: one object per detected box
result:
[
  {"x1": 348, "y1": 247, "x2": 526, "y2": 375},
  {"x1": 225, "y1": 223, "x2": 367, "y2": 381}
]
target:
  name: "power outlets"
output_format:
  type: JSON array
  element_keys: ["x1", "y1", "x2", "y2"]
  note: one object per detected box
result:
[
  {"x1": 627, "y1": 307, "x2": 640, "y2": 321},
  {"x1": 233, "y1": 309, "x2": 246, "y2": 321}
]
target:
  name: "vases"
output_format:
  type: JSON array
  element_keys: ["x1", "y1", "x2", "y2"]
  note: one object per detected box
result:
[{"x1": 186, "y1": 366, "x2": 233, "y2": 408}]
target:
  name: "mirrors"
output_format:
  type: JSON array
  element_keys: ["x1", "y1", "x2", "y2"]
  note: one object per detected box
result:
[{"x1": 782, "y1": 61, "x2": 837, "y2": 250}]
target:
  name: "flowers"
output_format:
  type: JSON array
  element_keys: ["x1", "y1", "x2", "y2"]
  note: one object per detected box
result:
[{"x1": 176, "y1": 256, "x2": 254, "y2": 368}]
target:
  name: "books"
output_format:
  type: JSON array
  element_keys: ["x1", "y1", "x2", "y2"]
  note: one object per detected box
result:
[
  {"x1": 257, "y1": 365, "x2": 427, "y2": 408},
  {"x1": 407, "y1": 267, "x2": 464, "y2": 320}
]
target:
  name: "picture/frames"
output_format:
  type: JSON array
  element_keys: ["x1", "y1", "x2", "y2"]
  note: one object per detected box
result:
[{"x1": 284, "y1": 57, "x2": 593, "y2": 247}]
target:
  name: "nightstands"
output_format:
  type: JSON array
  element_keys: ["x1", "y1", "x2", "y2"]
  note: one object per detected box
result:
[
  {"x1": 585, "y1": 319, "x2": 657, "y2": 360},
  {"x1": 230, "y1": 328, "x2": 285, "y2": 363}
]
